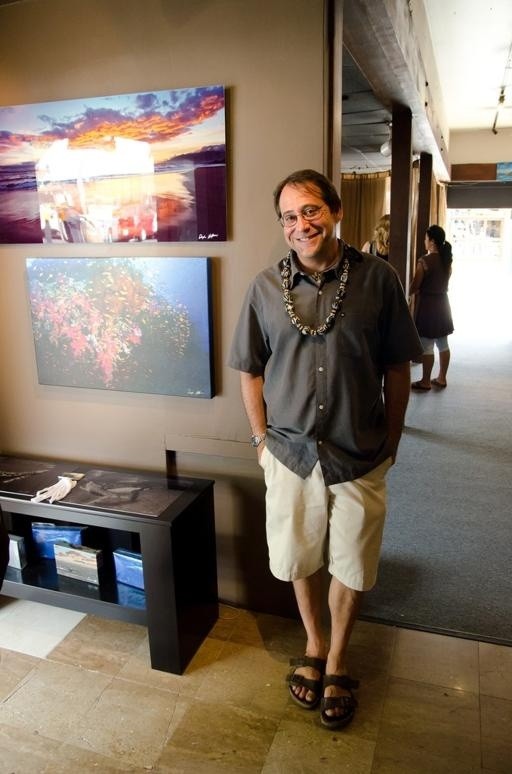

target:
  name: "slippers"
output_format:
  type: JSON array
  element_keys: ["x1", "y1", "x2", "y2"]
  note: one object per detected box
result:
[
  {"x1": 431, "y1": 378, "x2": 446, "y2": 387},
  {"x1": 319, "y1": 674, "x2": 359, "y2": 729},
  {"x1": 411, "y1": 381, "x2": 431, "y2": 389},
  {"x1": 285, "y1": 655, "x2": 326, "y2": 710}
]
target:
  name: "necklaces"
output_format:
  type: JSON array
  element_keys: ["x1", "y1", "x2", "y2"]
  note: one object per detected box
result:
[{"x1": 281, "y1": 242, "x2": 351, "y2": 337}]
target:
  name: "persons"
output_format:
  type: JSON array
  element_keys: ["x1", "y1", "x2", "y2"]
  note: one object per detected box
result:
[
  {"x1": 408, "y1": 226, "x2": 454, "y2": 389},
  {"x1": 229, "y1": 170, "x2": 424, "y2": 730},
  {"x1": 361, "y1": 214, "x2": 391, "y2": 262}
]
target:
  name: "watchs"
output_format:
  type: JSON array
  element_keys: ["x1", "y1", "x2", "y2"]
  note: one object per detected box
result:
[{"x1": 250, "y1": 434, "x2": 265, "y2": 446}]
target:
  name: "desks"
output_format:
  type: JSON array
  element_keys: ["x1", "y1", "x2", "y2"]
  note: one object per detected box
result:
[{"x1": 0, "y1": 456, "x2": 216, "y2": 674}]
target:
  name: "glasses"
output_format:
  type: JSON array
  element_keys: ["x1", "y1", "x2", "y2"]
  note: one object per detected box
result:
[{"x1": 277, "y1": 203, "x2": 325, "y2": 227}]
